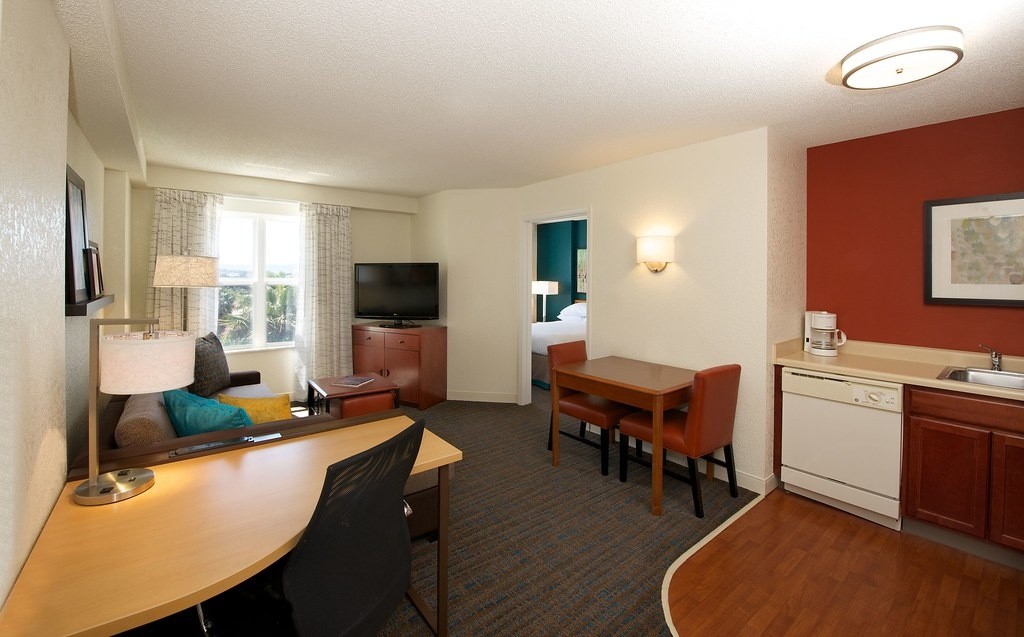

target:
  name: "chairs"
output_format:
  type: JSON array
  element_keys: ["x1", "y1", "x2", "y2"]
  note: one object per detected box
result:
[
  {"x1": 197, "y1": 419, "x2": 426, "y2": 637},
  {"x1": 547, "y1": 340, "x2": 643, "y2": 477},
  {"x1": 619, "y1": 364, "x2": 741, "y2": 518}
]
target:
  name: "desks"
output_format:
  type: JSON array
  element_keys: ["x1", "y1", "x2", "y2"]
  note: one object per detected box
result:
[
  {"x1": 0, "y1": 415, "x2": 462, "y2": 637},
  {"x1": 554, "y1": 356, "x2": 714, "y2": 515}
]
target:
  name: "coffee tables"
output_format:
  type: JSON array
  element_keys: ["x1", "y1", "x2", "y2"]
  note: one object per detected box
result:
[{"x1": 308, "y1": 372, "x2": 400, "y2": 416}]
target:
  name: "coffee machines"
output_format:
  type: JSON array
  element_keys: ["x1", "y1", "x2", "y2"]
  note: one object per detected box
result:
[{"x1": 804, "y1": 310, "x2": 846, "y2": 357}]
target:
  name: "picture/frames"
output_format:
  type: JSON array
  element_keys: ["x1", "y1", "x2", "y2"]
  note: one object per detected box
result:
[{"x1": 65, "y1": 163, "x2": 104, "y2": 305}]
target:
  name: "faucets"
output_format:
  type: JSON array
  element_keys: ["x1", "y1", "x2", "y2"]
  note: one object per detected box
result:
[{"x1": 979, "y1": 344, "x2": 1002, "y2": 371}]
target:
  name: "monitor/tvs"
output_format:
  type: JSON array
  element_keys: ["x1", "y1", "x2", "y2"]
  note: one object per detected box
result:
[{"x1": 354, "y1": 262, "x2": 439, "y2": 328}]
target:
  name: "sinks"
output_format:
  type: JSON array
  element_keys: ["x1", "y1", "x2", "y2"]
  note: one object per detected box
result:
[{"x1": 936, "y1": 366, "x2": 1024, "y2": 390}]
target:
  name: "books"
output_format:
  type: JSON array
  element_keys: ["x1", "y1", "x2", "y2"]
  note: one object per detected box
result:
[{"x1": 330, "y1": 375, "x2": 374, "y2": 388}]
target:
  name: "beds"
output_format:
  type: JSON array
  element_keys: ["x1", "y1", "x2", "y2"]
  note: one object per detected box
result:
[{"x1": 532, "y1": 299, "x2": 586, "y2": 389}]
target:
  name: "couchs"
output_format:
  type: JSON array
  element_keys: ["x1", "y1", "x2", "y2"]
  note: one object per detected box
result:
[{"x1": 71, "y1": 371, "x2": 334, "y2": 468}]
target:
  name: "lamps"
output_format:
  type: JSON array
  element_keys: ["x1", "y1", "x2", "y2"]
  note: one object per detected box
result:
[
  {"x1": 636, "y1": 236, "x2": 674, "y2": 273},
  {"x1": 532, "y1": 281, "x2": 558, "y2": 322},
  {"x1": 153, "y1": 254, "x2": 219, "y2": 331},
  {"x1": 73, "y1": 319, "x2": 194, "y2": 505}
]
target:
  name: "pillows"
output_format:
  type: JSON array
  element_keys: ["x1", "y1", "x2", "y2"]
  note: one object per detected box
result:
[
  {"x1": 557, "y1": 302, "x2": 586, "y2": 321},
  {"x1": 162, "y1": 389, "x2": 253, "y2": 438},
  {"x1": 217, "y1": 394, "x2": 293, "y2": 425},
  {"x1": 188, "y1": 332, "x2": 232, "y2": 397}
]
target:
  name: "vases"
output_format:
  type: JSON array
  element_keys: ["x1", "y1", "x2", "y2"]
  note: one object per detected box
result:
[{"x1": 923, "y1": 192, "x2": 1024, "y2": 306}]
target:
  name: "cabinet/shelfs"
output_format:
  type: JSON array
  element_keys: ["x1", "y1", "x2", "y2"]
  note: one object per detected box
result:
[
  {"x1": 352, "y1": 321, "x2": 447, "y2": 411},
  {"x1": 901, "y1": 384, "x2": 1024, "y2": 554}
]
